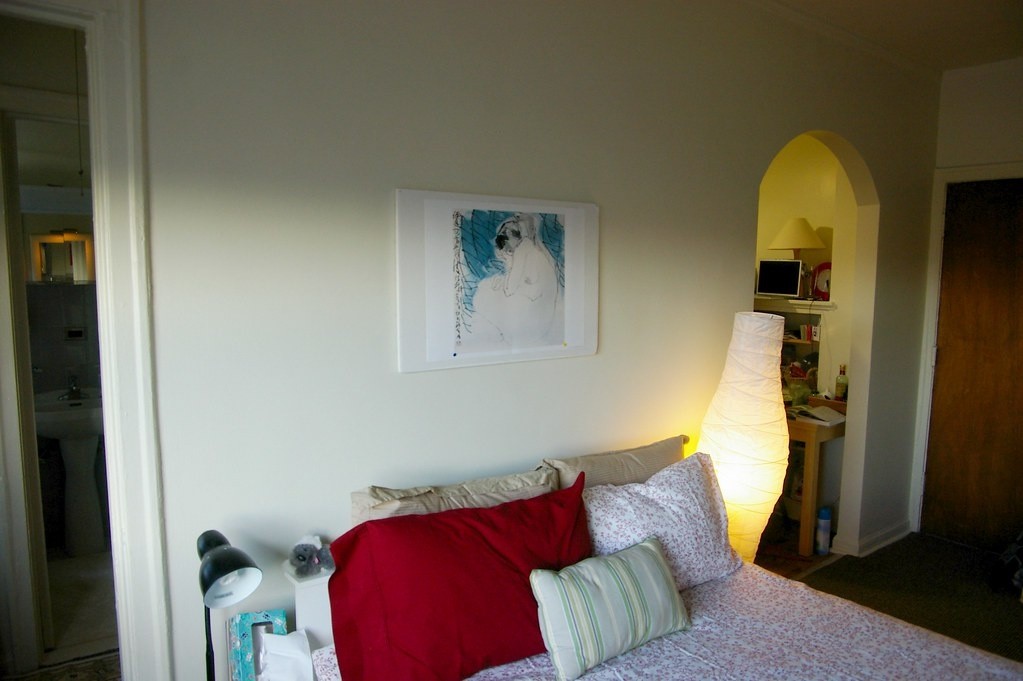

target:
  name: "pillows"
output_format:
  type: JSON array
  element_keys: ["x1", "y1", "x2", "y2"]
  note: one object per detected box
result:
[
  {"x1": 582, "y1": 451, "x2": 745, "y2": 591},
  {"x1": 529, "y1": 535, "x2": 693, "y2": 681},
  {"x1": 535, "y1": 435, "x2": 690, "y2": 489},
  {"x1": 328, "y1": 471, "x2": 590, "y2": 681},
  {"x1": 347, "y1": 462, "x2": 559, "y2": 528}
]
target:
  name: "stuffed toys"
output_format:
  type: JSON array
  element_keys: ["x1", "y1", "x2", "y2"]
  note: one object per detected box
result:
[{"x1": 289, "y1": 535, "x2": 335, "y2": 579}]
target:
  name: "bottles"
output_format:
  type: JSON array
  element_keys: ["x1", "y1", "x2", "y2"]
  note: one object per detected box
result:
[{"x1": 834, "y1": 364, "x2": 849, "y2": 401}]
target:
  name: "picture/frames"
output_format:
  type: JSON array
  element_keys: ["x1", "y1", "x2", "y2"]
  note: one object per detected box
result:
[{"x1": 395, "y1": 189, "x2": 598, "y2": 374}]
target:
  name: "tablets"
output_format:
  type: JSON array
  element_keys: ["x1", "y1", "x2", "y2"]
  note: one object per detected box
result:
[{"x1": 756, "y1": 259, "x2": 802, "y2": 297}]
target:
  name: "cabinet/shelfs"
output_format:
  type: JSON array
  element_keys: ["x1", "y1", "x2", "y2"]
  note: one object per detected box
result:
[{"x1": 754, "y1": 296, "x2": 835, "y2": 393}]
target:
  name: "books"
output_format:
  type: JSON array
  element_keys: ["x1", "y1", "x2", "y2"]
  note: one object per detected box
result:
[{"x1": 785, "y1": 405, "x2": 846, "y2": 427}]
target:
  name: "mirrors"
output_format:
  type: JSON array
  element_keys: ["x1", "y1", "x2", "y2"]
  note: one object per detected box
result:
[{"x1": 16, "y1": 118, "x2": 95, "y2": 284}]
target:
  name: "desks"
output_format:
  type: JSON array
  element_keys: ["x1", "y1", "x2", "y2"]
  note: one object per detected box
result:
[{"x1": 785, "y1": 400, "x2": 847, "y2": 559}]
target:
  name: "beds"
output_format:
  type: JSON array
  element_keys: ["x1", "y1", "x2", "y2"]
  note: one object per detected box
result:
[{"x1": 282, "y1": 434, "x2": 1023, "y2": 681}]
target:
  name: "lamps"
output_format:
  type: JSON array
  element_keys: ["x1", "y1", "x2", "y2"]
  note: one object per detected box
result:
[
  {"x1": 765, "y1": 217, "x2": 825, "y2": 300},
  {"x1": 196, "y1": 530, "x2": 263, "y2": 681},
  {"x1": 692, "y1": 312, "x2": 790, "y2": 565}
]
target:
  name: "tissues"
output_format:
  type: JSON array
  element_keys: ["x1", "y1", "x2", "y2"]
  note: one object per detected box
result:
[{"x1": 226, "y1": 608, "x2": 313, "y2": 681}]
